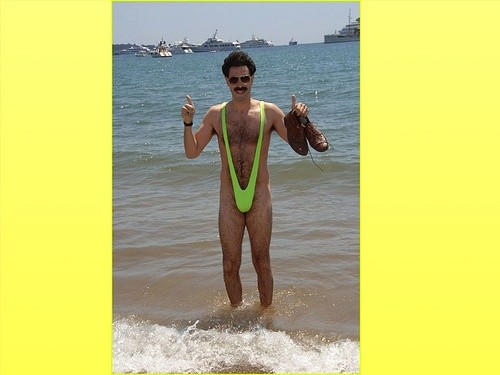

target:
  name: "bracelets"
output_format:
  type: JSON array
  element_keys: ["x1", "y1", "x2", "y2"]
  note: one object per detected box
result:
[{"x1": 184, "y1": 122, "x2": 194, "y2": 126}]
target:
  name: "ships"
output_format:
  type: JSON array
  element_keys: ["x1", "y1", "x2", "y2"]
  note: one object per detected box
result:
[{"x1": 323, "y1": 6, "x2": 360, "y2": 44}]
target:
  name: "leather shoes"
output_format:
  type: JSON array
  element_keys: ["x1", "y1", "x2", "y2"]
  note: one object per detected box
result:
[
  {"x1": 284, "y1": 110, "x2": 309, "y2": 156},
  {"x1": 300, "y1": 115, "x2": 328, "y2": 153}
]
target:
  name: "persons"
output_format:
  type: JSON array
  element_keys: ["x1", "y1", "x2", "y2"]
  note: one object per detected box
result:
[{"x1": 181, "y1": 52, "x2": 309, "y2": 307}]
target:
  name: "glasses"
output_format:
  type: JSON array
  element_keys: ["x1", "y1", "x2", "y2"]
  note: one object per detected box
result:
[{"x1": 229, "y1": 75, "x2": 251, "y2": 84}]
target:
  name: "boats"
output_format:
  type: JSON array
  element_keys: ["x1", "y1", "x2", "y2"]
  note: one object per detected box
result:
[
  {"x1": 288, "y1": 38, "x2": 297, "y2": 45},
  {"x1": 240, "y1": 33, "x2": 274, "y2": 47},
  {"x1": 150, "y1": 46, "x2": 173, "y2": 57},
  {"x1": 135, "y1": 49, "x2": 148, "y2": 57},
  {"x1": 113, "y1": 35, "x2": 194, "y2": 56},
  {"x1": 191, "y1": 27, "x2": 241, "y2": 52}
]
